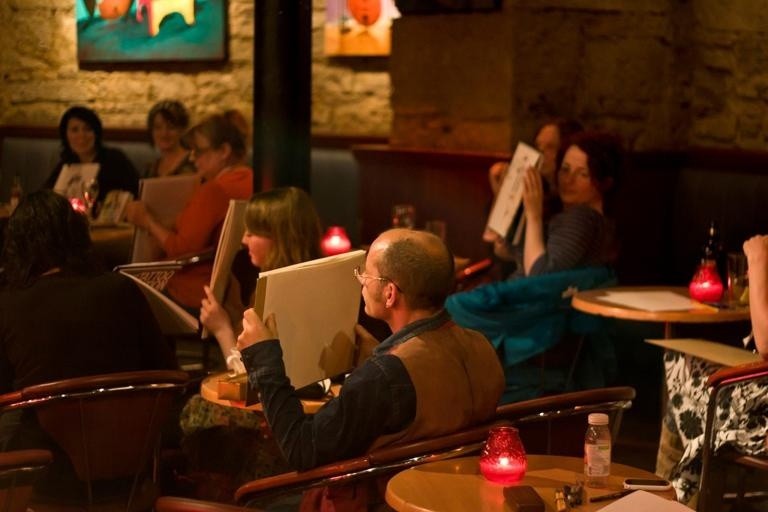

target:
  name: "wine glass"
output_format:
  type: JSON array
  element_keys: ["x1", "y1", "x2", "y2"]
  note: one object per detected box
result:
[{"x1": 82, "y1": 178, "x2": 101, "y2": 222}]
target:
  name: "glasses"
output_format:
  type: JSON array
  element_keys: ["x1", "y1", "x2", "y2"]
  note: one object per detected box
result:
[{"x1": 354, "y1": 266, "x2": 402, "y2": 293}]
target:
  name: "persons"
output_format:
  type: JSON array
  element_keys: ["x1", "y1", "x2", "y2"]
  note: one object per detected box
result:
[
  {"x1": 46, "y1": 105, "x2": 144, "y2": 208},
  {"x1": 499, "y1": 129, "x2": 618, "y2": 275},
  {"x1": 140, "y1": 97, "x2": 198, "y2": 186},
  {"x1": 2, "y1": 186, "x2": 172, "y2": 392},
  {"x1": 487, "y1": 115, "x2": 582, "y2": 232},
  {"x1": 194, "y1": 227, "x2": 508, "y2": 500},
  {"x1": 195, "y1": 186, "x2": 328, "y2": 380},
  {"x1": 656, "y1": 233, "x2": 767, "y2": 502},
  {"x1": 124, "y1": 110, "x2": 253, "y2": 313}
]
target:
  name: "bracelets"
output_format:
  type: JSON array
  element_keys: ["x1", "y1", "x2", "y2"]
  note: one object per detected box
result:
[{"x1": 224, "y1": 354, "x2": 236, "y2": 365}]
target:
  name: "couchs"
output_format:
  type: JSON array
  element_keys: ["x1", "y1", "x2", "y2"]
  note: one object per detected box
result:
[{"x1": 1, "y1": 138, "x2": 356, "y2": 237}]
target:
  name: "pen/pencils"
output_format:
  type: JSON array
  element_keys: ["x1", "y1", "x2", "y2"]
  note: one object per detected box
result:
[
  {"x1": 700, "y1": 301, "x2": 729, "y2": 308},
  {"x1": 589, "y1": 489, "x2": 637, "y2": 503}
]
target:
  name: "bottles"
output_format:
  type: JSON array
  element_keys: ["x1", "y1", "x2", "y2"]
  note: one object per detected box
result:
[
  {"x1": 582, "y1": 412, "x2": 611, "y2": 488},
  {"x1": 10, "y1": 175, "x2": 23, "y2": 211},
  {"x1": 687, "y1": 258, "x2": 725, "y2": 304},
  {"x1": 318, "y1": 228, "x2": 352, "y2": 257},
  {"x1": 479, "y1": 426, "x2": 526, "y2": 483}
]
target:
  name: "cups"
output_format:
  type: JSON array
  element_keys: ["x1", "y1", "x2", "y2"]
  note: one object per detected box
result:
[
  {"x1": 427, "y1": 220, "x2": 445, "y2": 241},
  {"x1": 393, "y1": 205, "x2": 417, "y2": 228}
]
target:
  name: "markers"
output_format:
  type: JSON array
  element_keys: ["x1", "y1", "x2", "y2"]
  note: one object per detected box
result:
[{"x1": 555, "y1": 487, "x2": 566, "y2": 511}]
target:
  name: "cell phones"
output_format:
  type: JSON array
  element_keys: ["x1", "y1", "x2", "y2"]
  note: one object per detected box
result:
[
  {"x1": 623, "y1": 479, "x2": 672, "y2": 491},
  {"x1": 502, "y1": 485, "x2": 545, "y2": 512}
]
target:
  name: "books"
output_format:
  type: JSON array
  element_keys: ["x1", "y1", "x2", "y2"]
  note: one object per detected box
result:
[
  {"x1": 252, "y1": 250, "x2": 371, "y2": 395},
  {"x1": 481, "y1": 140, "x2": 546, "y2": 246},
  {"x1": 129, "y1": 172, "x2": 203, "y2": 263},
  {"x1": 52, "y1": 162, "x2": 101, "y2": 197},
  {"x1": 201, "y1": 197, "x2": 249, "y2": 344}
]
